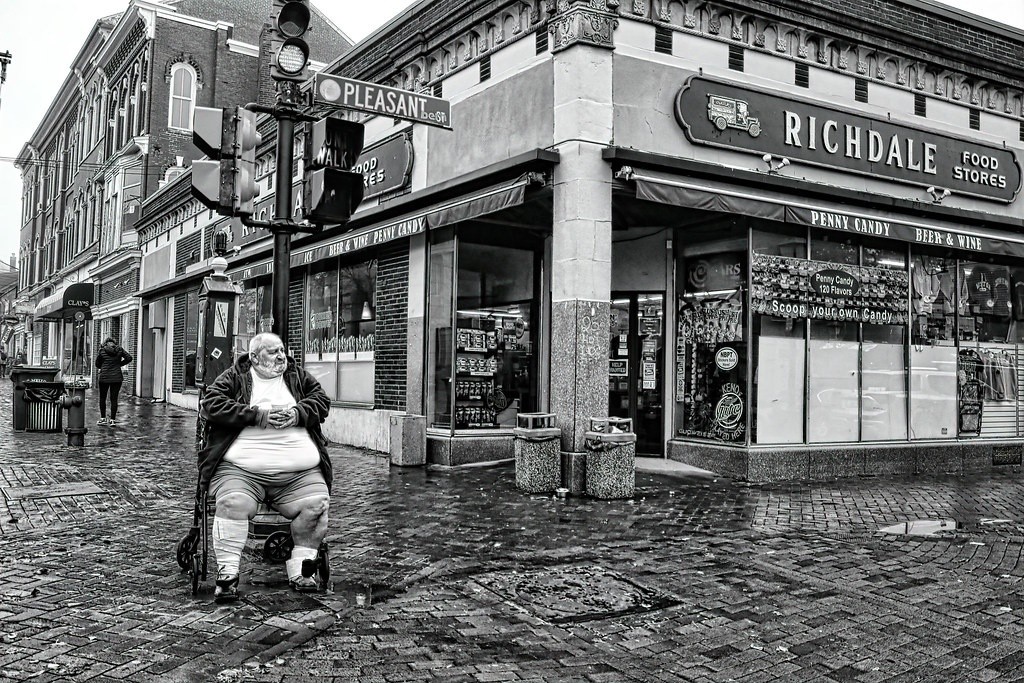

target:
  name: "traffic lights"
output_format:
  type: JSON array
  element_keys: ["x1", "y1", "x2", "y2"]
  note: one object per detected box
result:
[
  {"x1": 192, "y1": 104, "x2": 262, "y2": 217},
  {"x1": 308, "y1": 115, "x2": 366, "y2": 227},
  {"x1": 270, "y1": 0, "x2": 312, "y2": 82}
]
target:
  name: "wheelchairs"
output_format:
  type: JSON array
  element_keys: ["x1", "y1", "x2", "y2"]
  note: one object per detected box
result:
[{"x1": 177, "y1": 383, "x2": 331, "y2": 596}]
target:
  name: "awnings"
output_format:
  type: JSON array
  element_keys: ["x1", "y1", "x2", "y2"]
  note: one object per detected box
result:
[{"x1": 34, "y1": 282, "x2": 94, "y2": 323}]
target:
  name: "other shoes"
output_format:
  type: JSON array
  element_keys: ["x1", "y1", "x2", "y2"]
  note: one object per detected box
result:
[
  {"x1": 2, "y1": 373, "x2": 5, "y2": 377},
  {"x1": 290, "y1": 575, "x2": 317, "y2": 589},
  {"x1": 109, "y1": 419, "x2": 116, "y2": 427},
  {"x1": 214, "y1": 579, "x2": 239, "y2": 599},
  {"x1": 97, "y1": 418, "x2": 107, "y2": 426}
]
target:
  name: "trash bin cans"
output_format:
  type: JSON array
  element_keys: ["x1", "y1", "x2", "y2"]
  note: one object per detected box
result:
[
  {"x1": 584, "y1": 416, "x2": 638, "y2": 499},
  {"x1": 513, "y1": 411, "x2": 564, "y2": 493},
  {"x1": 23, "y1": 380, "x2": 65, "y2": 433},
  {"x1": 9, "y1": 365, "x2": 61, "y2": 430}
]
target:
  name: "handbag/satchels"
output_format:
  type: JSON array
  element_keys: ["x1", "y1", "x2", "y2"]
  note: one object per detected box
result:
[{"x1": 1, "y1": 352, "x2": 8, "y2": 361}]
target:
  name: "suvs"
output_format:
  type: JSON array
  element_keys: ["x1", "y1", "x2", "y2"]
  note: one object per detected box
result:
[{"x1": 828, "y1": 368, "x2": 1024, "y2": 439}]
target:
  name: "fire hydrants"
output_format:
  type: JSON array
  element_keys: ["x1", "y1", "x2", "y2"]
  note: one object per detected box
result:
[{"x1": 57, "y1": 376, "x2": 88, "y2": 447}]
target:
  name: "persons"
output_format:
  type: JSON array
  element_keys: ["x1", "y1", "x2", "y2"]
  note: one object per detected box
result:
[
  {"x1": 0, "y1": 346, "x2": 8, "y2": 378},
  {"x1": 202, "y1": 333, "x2": 331, "y2": 603},
  {"x1": 96, "y1": 338, "x2": 133, "y2": 426}
]
